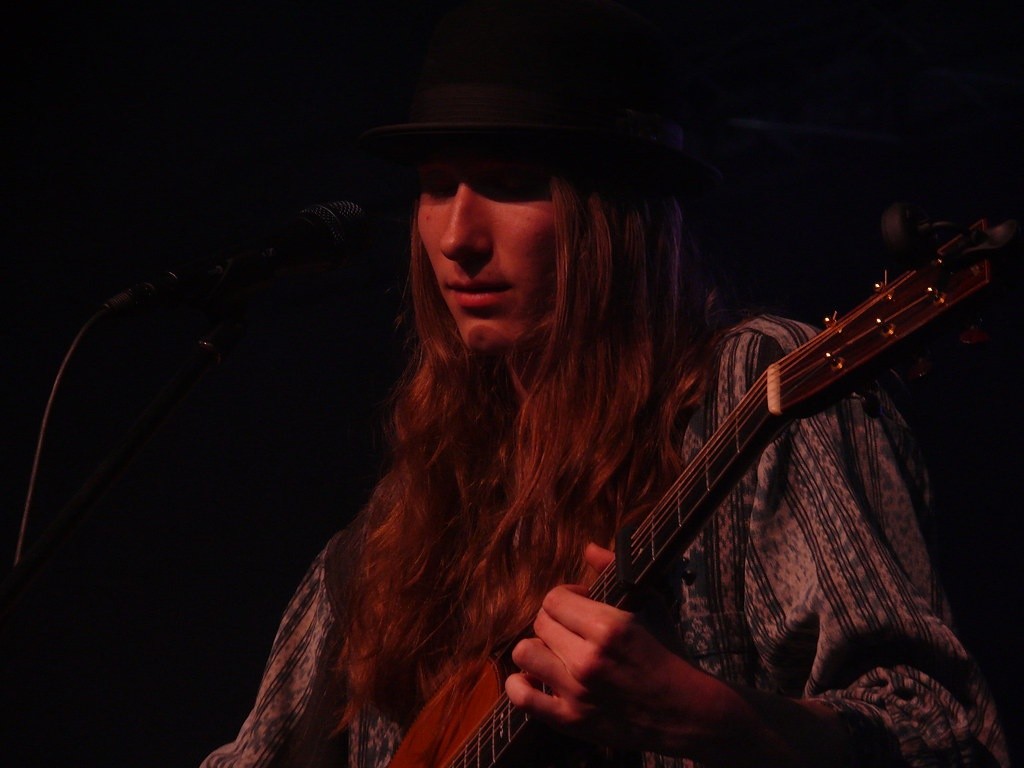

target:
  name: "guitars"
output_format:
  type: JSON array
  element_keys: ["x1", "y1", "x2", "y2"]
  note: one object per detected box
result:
[{"x1": 386, "y1": 210, "x2": 1024, "y2": 768}]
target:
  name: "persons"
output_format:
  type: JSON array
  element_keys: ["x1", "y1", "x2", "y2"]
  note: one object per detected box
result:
[{"x1": 198, "y1": 0, "x2": 1010, "y2": 767}]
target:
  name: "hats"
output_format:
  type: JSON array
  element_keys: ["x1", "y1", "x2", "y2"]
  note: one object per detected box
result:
[{"x1": 349, "y1": 3, "x2": 737, "y2": 184}]
